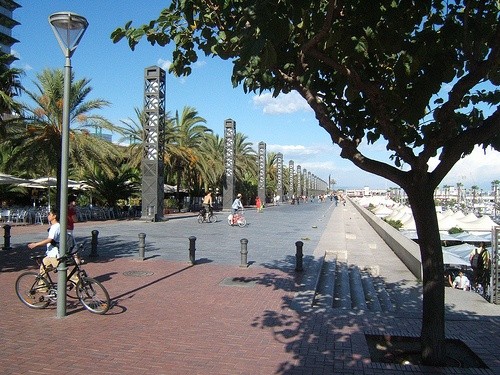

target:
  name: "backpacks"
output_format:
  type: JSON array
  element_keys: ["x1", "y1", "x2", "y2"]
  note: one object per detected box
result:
[{"x1": 471, "y1": 248, "x2": 488, "y2": 269}]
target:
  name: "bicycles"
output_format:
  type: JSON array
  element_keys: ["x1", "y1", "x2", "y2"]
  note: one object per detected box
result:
[
  {"x1": 197, "y1": 207, "x2": 217, "y2": 224},
  {"x1": 13, "y1": 238, "x2": 112, "y2": 314}
]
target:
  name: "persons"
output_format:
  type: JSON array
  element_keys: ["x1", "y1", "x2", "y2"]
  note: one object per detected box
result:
[
  {"x1": 452, "y1": 271, "x2": 469, "y2": 290},
  {"x1": 255, "y1": 196, "x2": 260, "y2": 213},
  {"x1": 319, "y1": 194, "x2": 324, "y2": 202},
  {"x1": 330, "y1": 194, "x2": 338, "y2": 207},
  {"x1": 292, "y1": 195, "x2": 314, "y2": 204},
  {"x1": 67, "y1": 194, "x2": 87, "y2": 265},
  {"x1": 202, "y1": 192, "x2": 213, "y2": 222},
  {"x1": 276, "y1": 194, "x2": 280, "y2": 206},
  {"x1": 28, "y1": 208, "x2": 80, "y2": 293},
  {"x1": 469, "y1": 242, "x2": 489, "y2": 292},
  {"x1": 231, "y1": 194, "x2": 244, "y2": 224}
]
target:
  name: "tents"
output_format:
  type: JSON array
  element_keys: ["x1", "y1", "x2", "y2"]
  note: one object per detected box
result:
[{"x1": 353, "y1": 196, "x2": 500, "y2": 266}]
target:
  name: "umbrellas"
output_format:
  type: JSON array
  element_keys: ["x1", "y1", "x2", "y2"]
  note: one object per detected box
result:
[{"x1": 0, "y1": 170, "x2": 102, "y2": 212}]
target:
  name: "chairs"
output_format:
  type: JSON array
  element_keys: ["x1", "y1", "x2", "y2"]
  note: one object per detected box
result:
[{"x1": 0, "y1": 204, "x2": 115, "y2": 225}]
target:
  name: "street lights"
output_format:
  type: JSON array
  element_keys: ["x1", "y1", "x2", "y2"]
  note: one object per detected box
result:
[{"x1": 45, "y1": 9, "x2": 90, "y2": 319}]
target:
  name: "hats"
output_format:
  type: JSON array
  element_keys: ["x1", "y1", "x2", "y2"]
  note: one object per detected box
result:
[
  {"x1": 68, "y1": 194, "x2": 77, "y2": 204},
  {"x1": 236, "y1": 193, "x2": 242, "y2": 199}
]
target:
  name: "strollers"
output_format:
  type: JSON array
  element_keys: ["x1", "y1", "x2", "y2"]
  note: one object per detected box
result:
[{"x1": 227, "y1": 208, "x2": 247, "y2": 228}]
